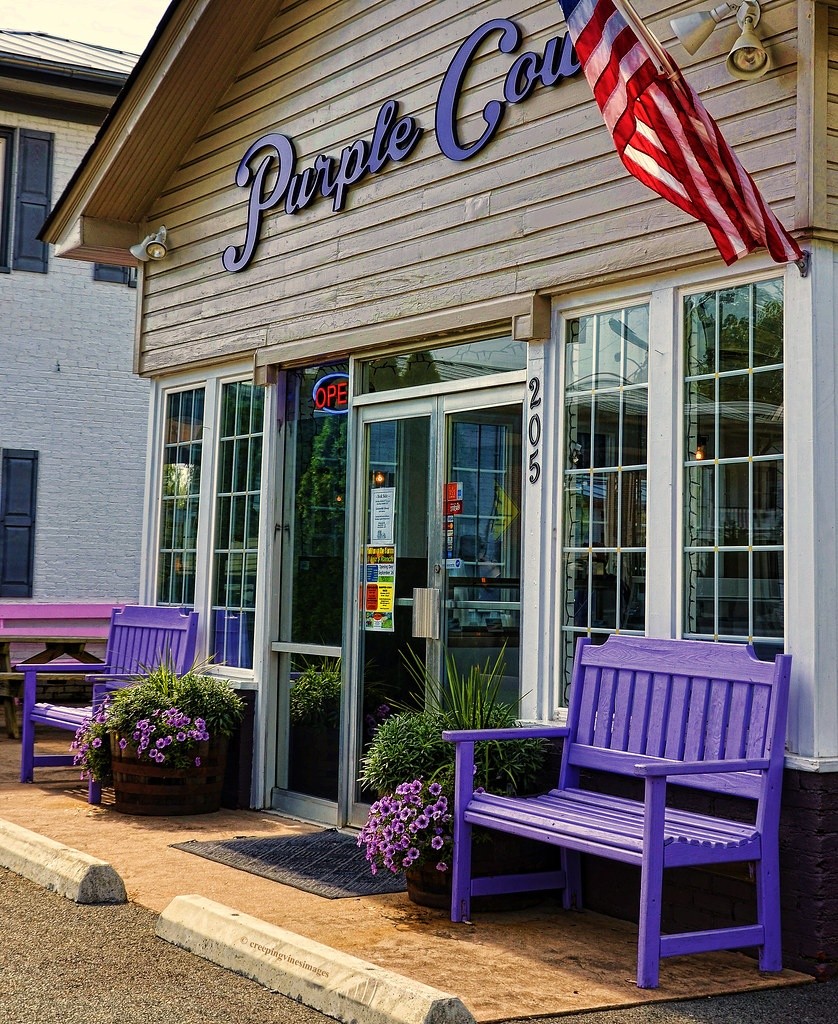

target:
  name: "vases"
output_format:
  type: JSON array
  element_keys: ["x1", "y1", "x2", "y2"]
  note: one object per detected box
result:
[
  {"x1": 110, "y1": 728, "x2": 229, "y2": 815},
  {"x1": 406, "y1": 837, "x2": 489, "y2": 909}
]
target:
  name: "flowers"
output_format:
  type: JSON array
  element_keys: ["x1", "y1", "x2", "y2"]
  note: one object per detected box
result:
[
  {"x1": 70, "y1": 648, "x2": 248, "y2": 782},
  {"x1": 357, "y1": 637, "x2": 553, "y2": 878}
]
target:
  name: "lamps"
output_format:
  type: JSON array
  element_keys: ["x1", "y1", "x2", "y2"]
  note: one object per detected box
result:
[
  {"x1": 127, "y1": 224, "x2": 167, "y2": 260},
  {"x1": 669, "y1": 0, "x2": 771, "y2": 81}
]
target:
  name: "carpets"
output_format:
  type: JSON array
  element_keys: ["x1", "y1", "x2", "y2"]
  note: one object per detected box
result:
[{"x1": 172, "y1": 828, "x2": 409, "y2": 900}]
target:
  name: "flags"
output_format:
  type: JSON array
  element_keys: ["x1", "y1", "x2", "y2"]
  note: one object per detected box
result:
[{"x1": 560, "y1": 0, "x2": 803, "y2": 267}]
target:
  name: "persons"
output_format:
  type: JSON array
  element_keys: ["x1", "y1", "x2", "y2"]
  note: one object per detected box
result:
[
  {"x1": 368, "y1": 554, "x2": 394, "y2": 564},
  {"x1": 575, "y1": 542, "x2": 630, "y2": 628}
]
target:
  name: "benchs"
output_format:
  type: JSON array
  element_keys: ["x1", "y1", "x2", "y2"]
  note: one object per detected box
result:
[
  {"x1": 16, "y1": 607, "x2": 198, "y2": 804},
  {"x1": 0, "y1": 601, "x2": 129, "y2": 739},
  {"x1": 442, "y1": 626, "x2": 792, "y2": 987}
]
